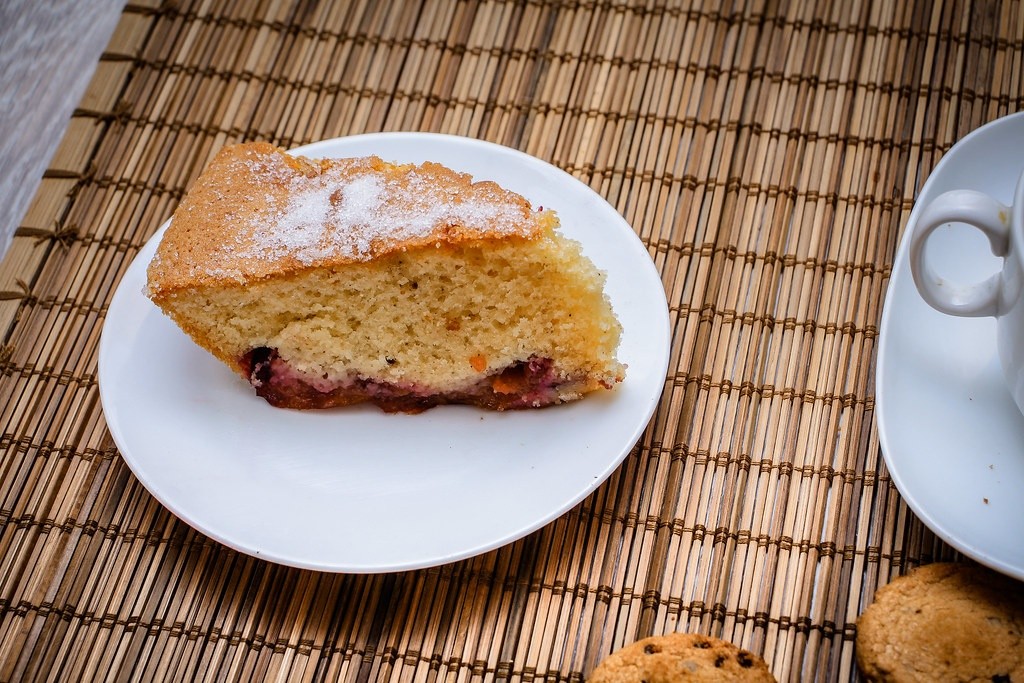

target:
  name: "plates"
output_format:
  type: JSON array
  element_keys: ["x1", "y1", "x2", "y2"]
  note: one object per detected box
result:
[
  {"x1": 874, "y1": 110, "x2": 1024, "y2": 580},
  {"x1": 98, "y1": 131, "x2": 672, "y2": 574}
]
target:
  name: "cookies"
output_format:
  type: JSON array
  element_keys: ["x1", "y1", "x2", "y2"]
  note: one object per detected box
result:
[
  {"x1": 585, "y1": 633, "x2": 778, "y2": 683},
  {"x1": 857, "y1": 562, "x2": 1024, "y2": 683}
]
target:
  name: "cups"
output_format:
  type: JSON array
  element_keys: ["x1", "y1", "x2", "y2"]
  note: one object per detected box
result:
[{"x1": 909, "y1": 171, "x2": 1024, "y2": 413}]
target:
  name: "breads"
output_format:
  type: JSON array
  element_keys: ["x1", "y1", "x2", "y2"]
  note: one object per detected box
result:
[{"x1": 142, "y1": 142, "x2": 632, "y2": 416}]
